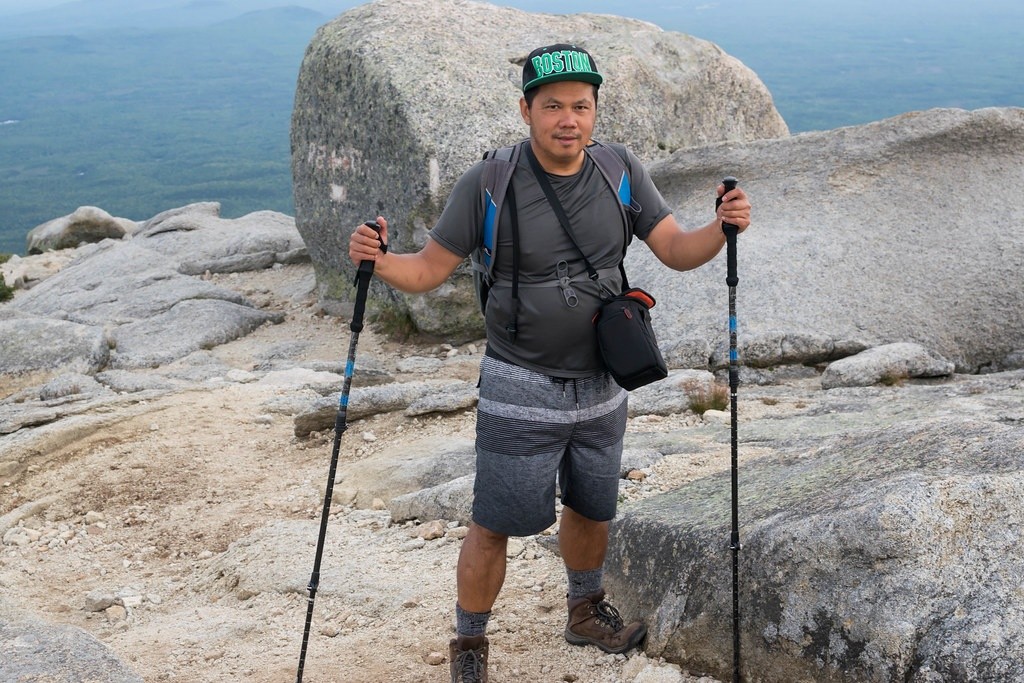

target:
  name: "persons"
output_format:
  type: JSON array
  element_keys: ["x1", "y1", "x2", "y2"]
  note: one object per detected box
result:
[{"x1": 349, "y1": 44, "x2": 751, "y2": 683}]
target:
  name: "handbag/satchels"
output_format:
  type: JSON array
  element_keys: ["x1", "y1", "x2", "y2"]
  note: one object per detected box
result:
[{"x1": 598, "y1": 287, "x2": 667, "y2": 392}]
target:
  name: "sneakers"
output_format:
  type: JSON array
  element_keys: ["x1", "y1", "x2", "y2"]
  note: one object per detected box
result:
[
  {"x1": 565, "y1": 588, "x2": 645, "y2": 653},
  {"x1": 447, "y1": 631, "x2": 489, "y2": 683}
]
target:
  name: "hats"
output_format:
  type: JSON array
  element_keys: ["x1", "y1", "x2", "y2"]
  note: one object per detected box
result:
[{"x1": 522, "y1": 43, "x2": 603, "y2": 94}]
